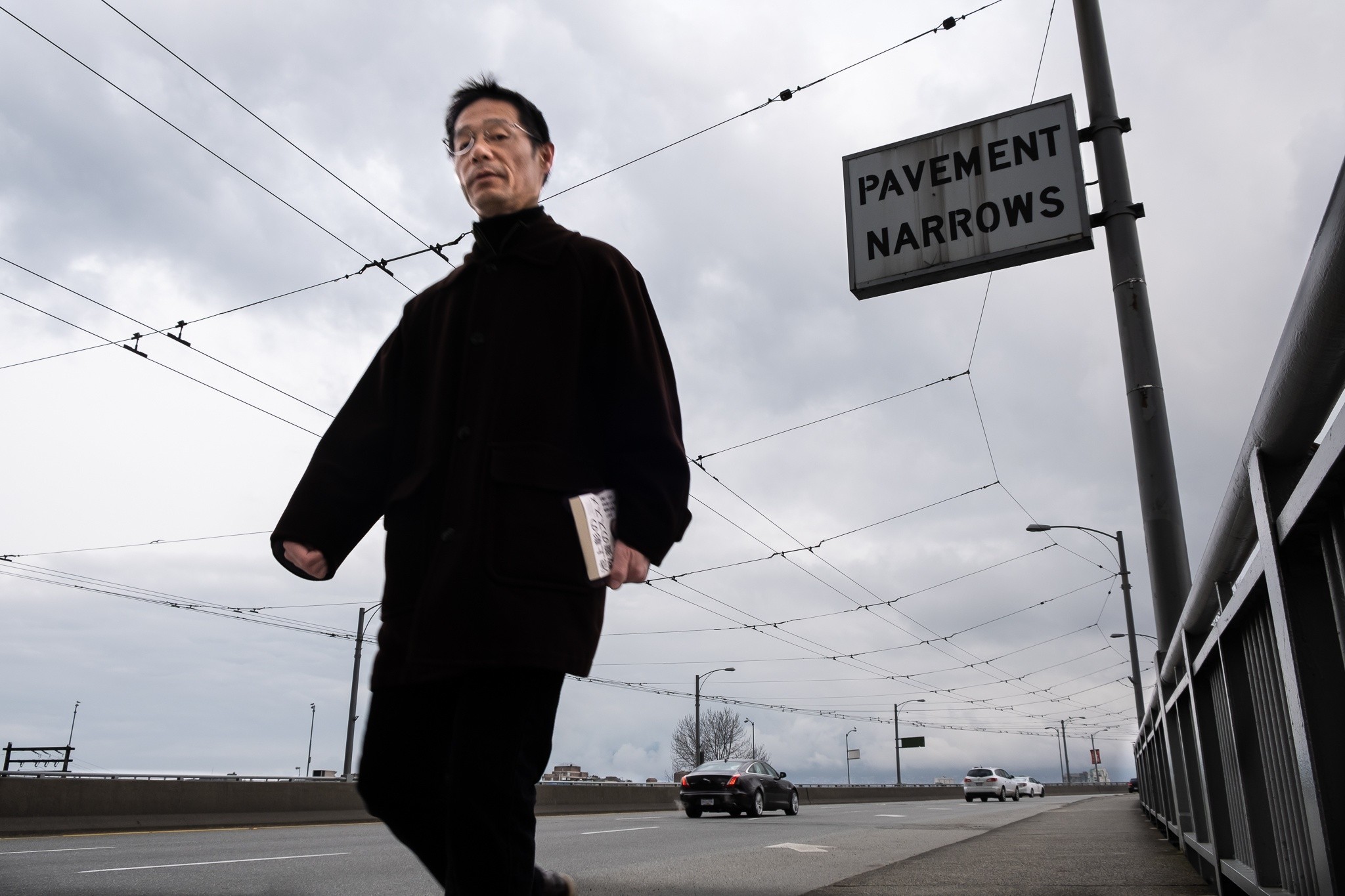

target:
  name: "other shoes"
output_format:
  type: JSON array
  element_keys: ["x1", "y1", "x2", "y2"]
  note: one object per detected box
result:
[{"x1": 542, "y1": 873, "x2": 575, "y2": 896}]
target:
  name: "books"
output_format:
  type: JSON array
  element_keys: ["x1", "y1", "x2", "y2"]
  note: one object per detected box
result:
[{"x1": 568, "y1": 489, "x2": 619, "y2": 581}]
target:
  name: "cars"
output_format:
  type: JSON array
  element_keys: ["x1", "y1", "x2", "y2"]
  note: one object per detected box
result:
[
  {"x1": 1014, "y1": 776, "x2": 1045, "y2": 798},
  {"x1": 1128, "y1": 778, "x2": 1139, "y2": 793},
  {"x1": 963, "y1": 766, "x2": 1019, "y2": 802},
  {"x1": 679, "y1": 759, "x2": 799, "y2": 818}
]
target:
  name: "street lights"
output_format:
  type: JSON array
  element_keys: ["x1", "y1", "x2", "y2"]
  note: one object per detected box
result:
[
  {"x1": 846, "y1": 730, "x2": 857, "y2": 786},
  {"x1": 1091, "y1": 729, "x2": 1108, "y2": 782},
  {"x1": 1026, "y1": 524, "x2": 1145, "y2": 730},
  {"x1": 1044, "y1": 727, "x2": 1064, "y2": 782},
  {"x1": 894, "y1": 699, "x2": 926, "y2": 785},
  {"x1": 1061, "y1": 717, "x2": 1086, "y2": 782},
  {"x1": 695, "y1": 668, "x2": 736, "y2": 767},
  {"x1": 744, "y1": 720, "x2": 755, "y2": 760}
]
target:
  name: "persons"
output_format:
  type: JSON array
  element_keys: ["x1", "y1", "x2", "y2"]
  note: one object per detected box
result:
[{"x1": 268, "y1": 81, "x2": 689, "y2": 896}]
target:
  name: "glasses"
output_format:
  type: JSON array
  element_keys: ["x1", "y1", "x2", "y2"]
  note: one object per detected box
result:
[{"x1": 442, "y1": 119, "x2": 544, "y2": 156}]
